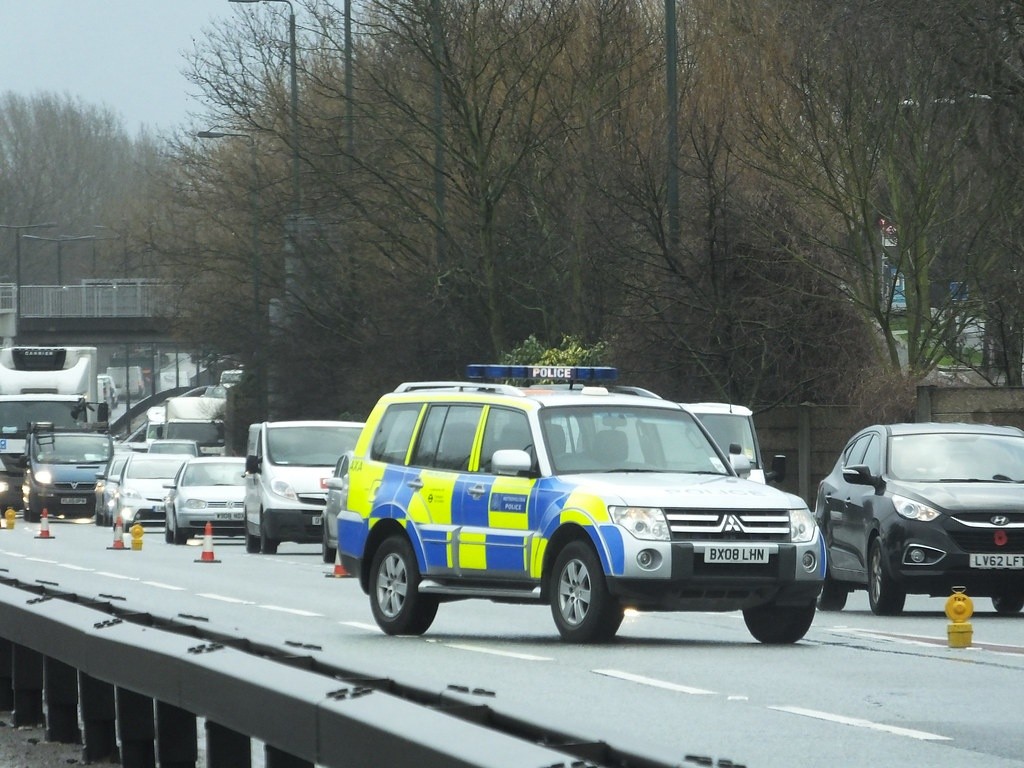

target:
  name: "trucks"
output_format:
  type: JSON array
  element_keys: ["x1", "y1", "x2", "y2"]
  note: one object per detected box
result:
[
  {"x1": 0, "y1": 345, "x2": 98, "y2": 512},
  {"x1": 144, "y1": 407, "x2": 163, "y2": 442},
  {"x1": 156, "y1": 397, "x2": 227, "y2": 456}
]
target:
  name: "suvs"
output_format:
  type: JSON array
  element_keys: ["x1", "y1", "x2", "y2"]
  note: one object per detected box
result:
[{"x1": 338, "y1": 359, "x2": 829, "y2": 644}]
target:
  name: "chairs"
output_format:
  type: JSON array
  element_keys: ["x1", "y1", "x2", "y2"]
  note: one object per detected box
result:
[{"x1": 534, "y1": 419, "x2": 630, "y2": 475}]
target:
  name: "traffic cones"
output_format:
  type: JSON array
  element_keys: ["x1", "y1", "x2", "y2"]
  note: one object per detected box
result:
[
  {"x1": 323, "y1": 544, "x2": 354, "y2": 577},
  {"x1": 106, "y1": 515, "x2": 131, "y2": 550},
  {"x1": 194, "y1": 521, "x2": 222, "y2": 563},
  {"x1": 34, "y1": 506, "x2": 55, "y2": 539}
]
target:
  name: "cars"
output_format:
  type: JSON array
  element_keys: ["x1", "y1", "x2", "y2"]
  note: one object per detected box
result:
[
  {"x1": 322, "y1": 453, "x2": 354, "y2": 564},
  {"x1": 108, "y1": 452, "x2": 195, "y2": 533},
  {"x1": 93, "y1": 453, "x2": 128, "y2": 527},
  {"x1": 814, "y1": 422, "x2": 1024, "y2": 617},
  {"x1": 161, "y1": 457, "x2": 247, "y2": 545}
]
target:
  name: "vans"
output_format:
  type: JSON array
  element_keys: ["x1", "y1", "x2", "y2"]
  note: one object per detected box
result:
[
  {"x1": 148, "y1": 439, "x2": 201, "y2": 457},
  {"x1": 97, "y1": 374, "x2": 120, "y2": 416},
  {"x1": 219, "y1": 370, "x2": 241, "y2": 391},
  {"x1": 244, "y1": 421, "x2": 366, "y2": 553},
  {"x1": 107, "y1": 366, "x2": 145, "y2": 400},
  {"x1": 19, "y1": 430, "x2": 114, "y2": 524},
  {"x1": 548, "y1": 403, "x2": 787, "y2": 485}
]
